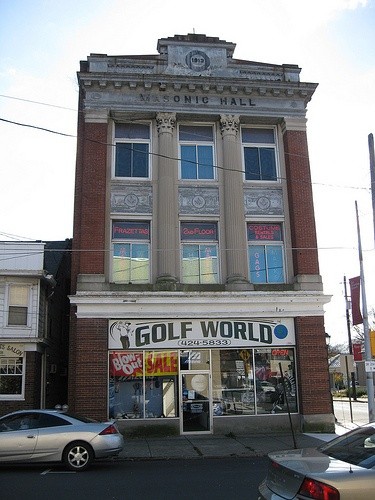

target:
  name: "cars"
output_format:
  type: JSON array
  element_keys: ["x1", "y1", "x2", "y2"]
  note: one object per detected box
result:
[
  {"x1": 259, "y1": 381, "x2": 275, "y2": 393},
  {"x1": 0, "y1": 410, "x2": 124, "y2": 472},
  {"x1": 256, "y1": 420, "x2": 375, "y2": 500}
]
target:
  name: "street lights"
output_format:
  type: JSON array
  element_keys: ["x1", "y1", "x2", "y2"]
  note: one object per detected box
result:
[{"x1": 324, "y1": 333, "x2": 337, "y2": 424}]
